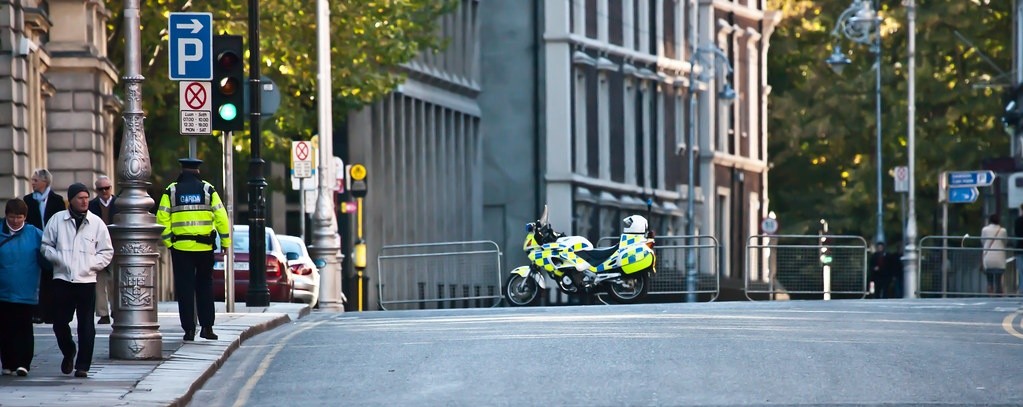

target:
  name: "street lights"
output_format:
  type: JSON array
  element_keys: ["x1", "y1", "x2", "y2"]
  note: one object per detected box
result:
[
  {"x1": 825, "y1": 7, "x2": 883, "y2": 247},
  {"x1": 682, "y1": 49, "x2": 736, "y2": 303}
]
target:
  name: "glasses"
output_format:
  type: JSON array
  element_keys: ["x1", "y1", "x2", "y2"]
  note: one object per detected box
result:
[{"x1": 97, "y1": 185, "x2": 111, "y2": 192}]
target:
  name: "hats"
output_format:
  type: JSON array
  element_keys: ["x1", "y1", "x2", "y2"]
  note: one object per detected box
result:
[
  {"x1": 68, "y1": 184, "x2": 90, "y2": 201},
  {"x1": 178, "y1": 158, "x2": 204, "y2": 169}
]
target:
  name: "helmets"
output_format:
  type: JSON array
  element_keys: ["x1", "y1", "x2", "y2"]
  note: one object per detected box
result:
[{"x1": 623, "y1": 215, "x2": 648, "y2": 234}]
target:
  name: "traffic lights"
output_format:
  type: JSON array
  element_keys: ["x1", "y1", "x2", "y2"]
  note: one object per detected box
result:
[{"x1": 213, "y1": 35, "x2": 244, "y2": 132}]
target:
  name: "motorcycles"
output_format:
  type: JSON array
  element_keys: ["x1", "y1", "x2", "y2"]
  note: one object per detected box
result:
[{"x1": 503, "y1": 204, "x2": 658, "y2": 307}]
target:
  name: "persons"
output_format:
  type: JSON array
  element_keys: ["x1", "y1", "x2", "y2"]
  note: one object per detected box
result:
[
  {"x1": 156, "y1": 159, "x2": 231, "y2": 341},
  {"x1": 0, "y1": 197, "x2": 53, "y2": 376},
  {"x1": 22, "y1": 168, "x2": 66, "y2": 323},
  {"x1": 866, "y1": 203, "x2": 1023, "y2": 299},
  {"x1": 39, "y1": 183, "x2": 114, "y2": 377},
  {"x1": 88, "y1": 175, "x2": 117, "y2": 324}
]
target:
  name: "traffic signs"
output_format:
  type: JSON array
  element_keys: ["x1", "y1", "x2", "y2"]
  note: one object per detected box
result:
[
  {"x1": 949, "y1": 187, "x2": 980, "y2": 202},
  {"x1": 169, "y1": 12, "x2": 212, "y2": 81},
  {"x1": 946, "y1": 169, "x2": 995, "y2": 187}
]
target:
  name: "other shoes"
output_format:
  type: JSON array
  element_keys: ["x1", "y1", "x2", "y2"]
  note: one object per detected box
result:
[
  {"x1": 75, "y1": 368, "x2": 88, "y2": 377},
  {"x1": 2, "y1": 369, "x2": 12, "y2": 375},
  {"x1": 97, "y1": 316, "x2": 110, "y2": 324},
  {"x1": 200, "y1": 326, "x2": 218, "y2": 340},
  {"x1": 16, "y1": 367, "x2": 28, "y2": 376},
  {"x1": 60, "y1": 349, "x2": 76, "y2": 374},
  {"x1": 183, "y1": 328, "x2": 195, "y2": 341}
]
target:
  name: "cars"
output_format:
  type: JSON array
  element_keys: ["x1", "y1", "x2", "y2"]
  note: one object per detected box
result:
[
  {"x1": 274, "y1": 234, "x2": 324, "y2": 309},
  {"x1": 210, "y1": 225, "x2": 298, "y2": 303}
]
